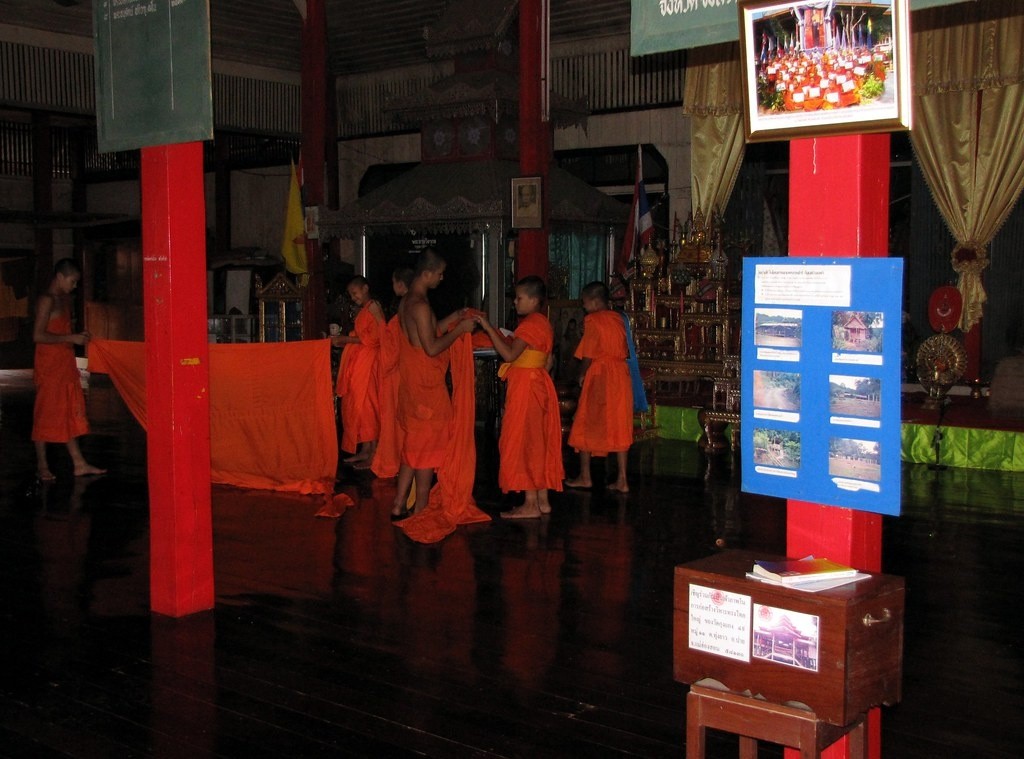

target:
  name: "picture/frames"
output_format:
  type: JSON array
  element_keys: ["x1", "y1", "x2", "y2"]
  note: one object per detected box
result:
[
  {"x1": 738, "y1": 0, "x2": 913, "y2": 144},
  {"x1": 509, "y1": 175, "x2": 544, "y2": 230}
]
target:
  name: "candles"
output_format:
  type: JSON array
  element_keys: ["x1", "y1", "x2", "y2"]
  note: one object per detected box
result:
[
  {"x1": 651, "y1": 290, "x2": 655, "y2": 311},
  {"x1": 680, "y1": 290, "x2": 684, "y2": 314}
]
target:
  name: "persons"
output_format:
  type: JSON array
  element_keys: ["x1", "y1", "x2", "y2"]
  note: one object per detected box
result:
[
  {"x1": 327, "y1": 319, "x2": 343, "y2": 416},
  {"x1": 766, "y1": 47, "x2": 886, "y2": 111},
  {"x1": 369, "y1": 267, "x2": 415, "y2": 481},
  {"x1": 472, "y1": 275, "x2": 565, "y2": 519},
  {"x1": 31, "y1": 257, "x2": 107, "y2": 481},
  {"x1": 564, "y1": 280, "x2": 633, "y2": 493},
  {"x1": 517, "y1": 186, "x2": 538, "y2": 219},
  {"x1": 332, "y1": 274, "x2": 386, "y2": 470},
  {"x1": 391, "y1": 245, "x2": 477, "y2": 517}
]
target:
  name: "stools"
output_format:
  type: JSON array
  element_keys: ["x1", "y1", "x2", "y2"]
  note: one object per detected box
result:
[{"x1": 686, "y1": 681, "x2": 867, "y2": 759}]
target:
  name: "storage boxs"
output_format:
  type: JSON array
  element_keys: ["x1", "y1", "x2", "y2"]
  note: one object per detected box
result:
[{"x1": 671, "y1": 551, "x2": 907, "y2": 727}]
